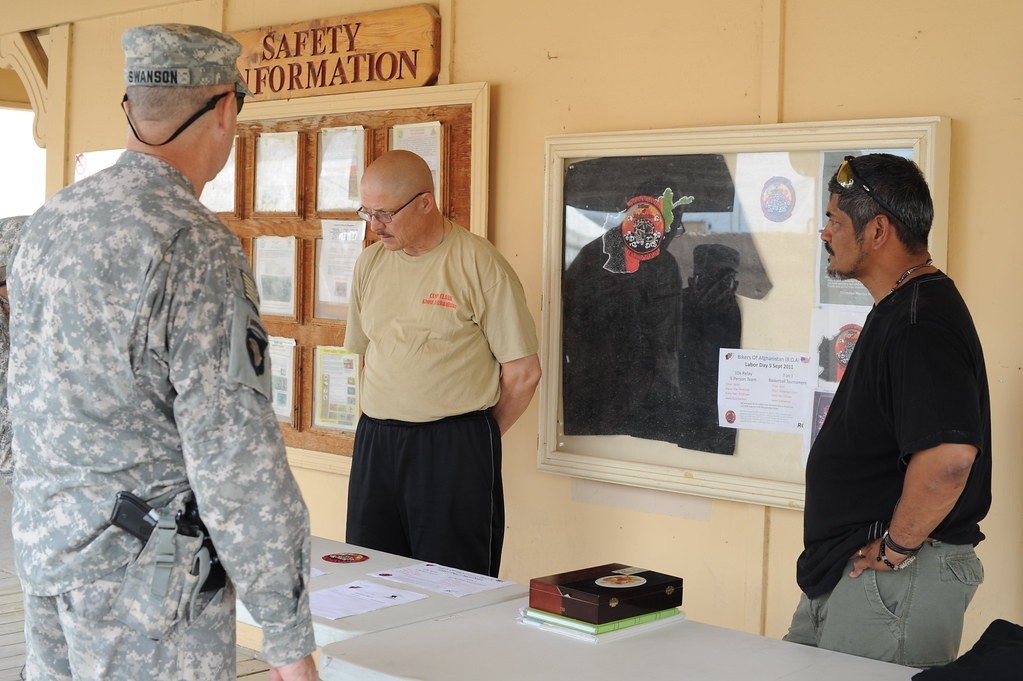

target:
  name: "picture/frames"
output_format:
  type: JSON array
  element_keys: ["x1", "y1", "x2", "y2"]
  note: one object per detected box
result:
[{"x1": 534, "y1": 113, "x2": 953, "y2": 509}]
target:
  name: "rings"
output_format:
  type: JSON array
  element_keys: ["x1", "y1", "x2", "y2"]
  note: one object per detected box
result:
[{"x1": 859, "y1": 550, "x2": 864, "y2": 558}]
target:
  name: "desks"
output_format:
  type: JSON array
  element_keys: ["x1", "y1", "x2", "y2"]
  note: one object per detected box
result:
[
  {"x1": 317, "y1": 554, "x2": 925, "y2": 681},
  {"x1": 236, "y1": 535, "x2": 527, "y2": 648}
]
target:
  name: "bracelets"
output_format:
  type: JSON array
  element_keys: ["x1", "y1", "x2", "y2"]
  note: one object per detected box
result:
[
  {"x1": 884, "y1": 523, "x2": 924, "y2": 553},
  {"x1": 877, "y1": 540, "x2": 915, "y2": 570}
]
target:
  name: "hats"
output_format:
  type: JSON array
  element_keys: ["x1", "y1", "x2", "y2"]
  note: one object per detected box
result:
[
  {"x1": 692, "y1": 245, "x2": 740, "y2": 274},
  {"x1": 121, "y1": 24, "x2": 256, "y2": 101}
]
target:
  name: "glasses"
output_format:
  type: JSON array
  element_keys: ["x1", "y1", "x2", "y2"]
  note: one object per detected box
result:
[
  {"x1": 232, "y1": 91, "x2": 246, "y2": 114},
  {"x1": 355, "y1": 191, "x2": 431, "y2": 224},
  {"x1": 835, "y1": 156, "x2": 908, "y2": 227},
  {"x1": 690, "y1": 271, "x2": 736, "y2": 291},
  {"x1": 672, "y1": 210, "x2": 686, "y2": 239}
]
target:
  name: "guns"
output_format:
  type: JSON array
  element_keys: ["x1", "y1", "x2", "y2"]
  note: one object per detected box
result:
[{"x1": 111, "y1": 490, "x2": 200, "y2": 577}]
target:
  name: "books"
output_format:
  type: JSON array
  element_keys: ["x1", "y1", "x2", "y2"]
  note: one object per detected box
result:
[{"x1": 522, "y1": 608, "x2": 685, "y2": 642}]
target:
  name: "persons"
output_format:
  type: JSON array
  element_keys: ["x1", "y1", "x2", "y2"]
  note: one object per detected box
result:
[
  {"x1": 783, "y1": 152, "x2": 992, "y2": 666},
  {"x1": 344, "y1": 150, "x2": 542, "y2": 578},
  {"x1": 0, "y1": 23, "x2": 322, "y2": 681},
  {"x1": 560, "y1": 176, "x2": 742, "y2": 457}
]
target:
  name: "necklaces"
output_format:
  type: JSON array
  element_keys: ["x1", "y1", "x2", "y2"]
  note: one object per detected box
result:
[{"x1": 889, "y1": 259, "x2": 932, "y2": 292}]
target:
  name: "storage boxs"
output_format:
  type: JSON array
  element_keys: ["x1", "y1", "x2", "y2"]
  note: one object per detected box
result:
[{"x1": 530, "y1": 561, "x2": 684, "y2": 624}]
